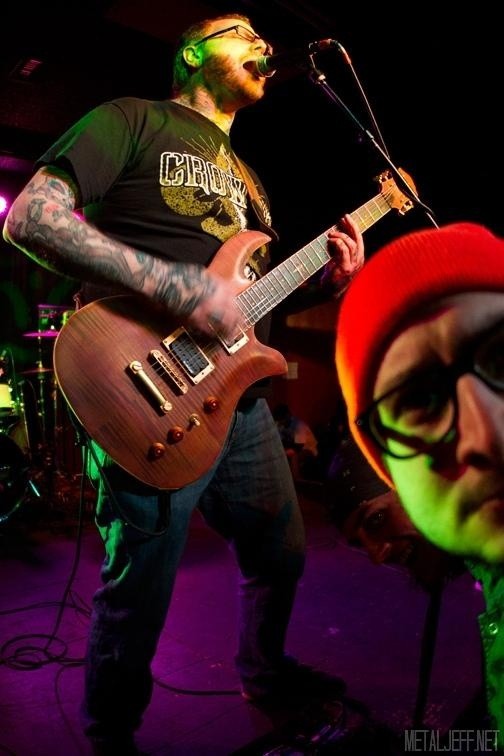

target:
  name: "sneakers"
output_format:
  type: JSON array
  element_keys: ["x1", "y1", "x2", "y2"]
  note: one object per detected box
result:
[{"x1": 242, "y1": 660, "x2": 347, "y2": 707}]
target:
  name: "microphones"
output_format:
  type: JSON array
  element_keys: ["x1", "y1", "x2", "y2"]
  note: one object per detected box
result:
[{"x1": 254, "y1": 38, "x2": 332, "y2": 79}]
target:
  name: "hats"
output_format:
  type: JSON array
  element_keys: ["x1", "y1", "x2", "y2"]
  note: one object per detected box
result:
[{"x1": 332, "y1": 218, "x2": 503, "y2": 489}]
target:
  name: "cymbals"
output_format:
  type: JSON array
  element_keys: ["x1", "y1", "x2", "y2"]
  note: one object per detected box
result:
[
  {"x1": 19, "y1": 368, "x2": 53, "y2": 375},
  {"x1": 24, "y1": 330, "x2": 59, "y2": 337}
]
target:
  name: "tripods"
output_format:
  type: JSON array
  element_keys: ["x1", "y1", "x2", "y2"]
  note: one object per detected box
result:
[{"x1": 24, "y1": 336, "x2": 83, "y2": 518}]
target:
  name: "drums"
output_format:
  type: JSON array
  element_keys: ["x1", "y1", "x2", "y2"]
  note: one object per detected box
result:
[{"x1": 0, "y1": 432, "x2": 33, "y2": 522}]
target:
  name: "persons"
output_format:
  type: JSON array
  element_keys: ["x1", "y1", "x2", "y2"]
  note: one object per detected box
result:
[
  {"x1": 322, "y1": 437, "x2": 479, "y2": 595},
  {"x1": 270, "y1": 404, "x2": 318, "y2": 463},
  {"x1": 1, "y1": 5, "x2": 367, "y2": 755},
  {"x1": 331, "y1": 222, "x2": 503, "y2": 752}
]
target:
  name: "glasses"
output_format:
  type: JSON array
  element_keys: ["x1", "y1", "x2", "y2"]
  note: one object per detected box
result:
[
  {"x1": 196, "y1": 23, "x2": 275, "y2": 58},
  {"x1": 354, "y1": 317, "x2": 501, "y2": 464}
]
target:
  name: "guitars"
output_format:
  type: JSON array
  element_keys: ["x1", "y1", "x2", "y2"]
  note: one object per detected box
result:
[{"x1": 52, "y1": 165, "x2": 417, "y2": 491}]
